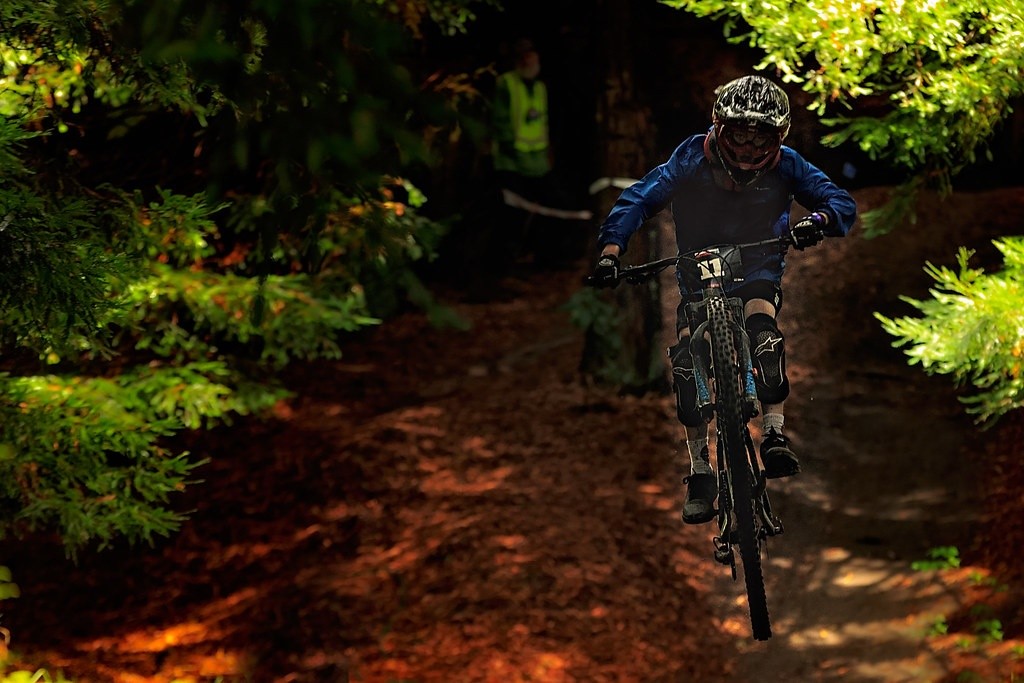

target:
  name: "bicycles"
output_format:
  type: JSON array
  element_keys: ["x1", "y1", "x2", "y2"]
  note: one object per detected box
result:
[{"x1": 583, "y1": 230, "x2": 802, "y2": 644}]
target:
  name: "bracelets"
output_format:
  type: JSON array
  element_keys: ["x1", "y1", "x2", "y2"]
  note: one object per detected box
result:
[{"x1": 809, "y1": 211, "x2": 822, "y2": 223}]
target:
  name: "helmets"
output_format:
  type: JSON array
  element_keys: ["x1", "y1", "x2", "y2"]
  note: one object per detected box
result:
[{"x1": 712, "y1": 75, "x2": 791, "y2": 187}]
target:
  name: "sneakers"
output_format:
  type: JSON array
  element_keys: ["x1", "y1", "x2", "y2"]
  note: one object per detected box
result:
[
  {"x1": 759, "y1": 425, "x2": 798, "y2": 478},
  {"x1": 682, "y1": 473, "x2": 718, "y2": 524}
]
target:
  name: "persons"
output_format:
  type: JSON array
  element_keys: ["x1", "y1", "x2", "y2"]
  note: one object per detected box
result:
[
  {"x1": 490, "y1": 50, "x2": 556, "y2": 260},
  {"x1": 592, "y1": 74, "x2": 856, "y2": 523}
]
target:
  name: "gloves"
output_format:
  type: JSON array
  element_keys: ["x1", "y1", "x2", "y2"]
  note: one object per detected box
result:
[
  {"x1": 593, "y1": 253, "x2": 622, "y2": 291},
  {"x1": 790, "y1": 219, "x2": 821, "y2": 252}
]
